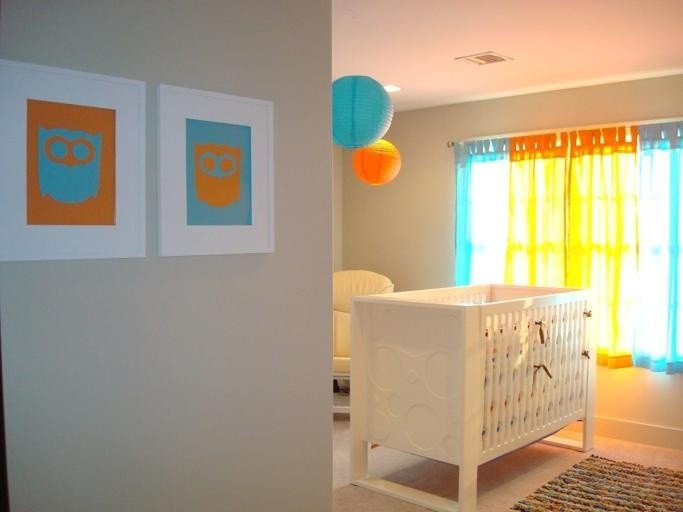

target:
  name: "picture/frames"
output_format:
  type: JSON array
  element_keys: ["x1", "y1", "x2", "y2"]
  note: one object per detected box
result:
[
  {"x1": 1, "y1": 56, "x2": 150, "y2": 264},
  {"x1": 154, "y1": 82, "x2": 274, "y2": 260}
]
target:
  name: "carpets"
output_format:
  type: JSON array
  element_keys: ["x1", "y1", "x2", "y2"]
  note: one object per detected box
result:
[{"x1": 509, "y1": 454, "x2": 683, "y2": 512}]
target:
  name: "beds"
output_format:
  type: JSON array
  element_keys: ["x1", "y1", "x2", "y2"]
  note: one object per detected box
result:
[{"x1": 347, "y1": 283, "x2": 594, "y2": 512}]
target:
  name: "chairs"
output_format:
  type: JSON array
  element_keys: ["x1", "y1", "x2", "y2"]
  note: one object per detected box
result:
[{"x1": 332, "y1": 268, "x2": 395, "y2": 415}]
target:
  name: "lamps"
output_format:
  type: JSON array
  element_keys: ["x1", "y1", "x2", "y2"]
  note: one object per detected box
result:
[{"x1": 331, "y1": 73, "x2": 402, "y2": 187}]
